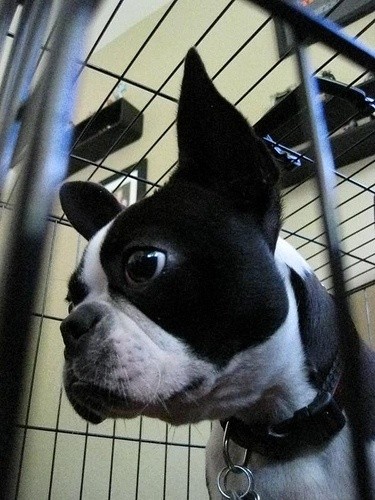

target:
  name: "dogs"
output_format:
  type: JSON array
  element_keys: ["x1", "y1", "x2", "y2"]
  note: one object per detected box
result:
[{"x1": 59, "y1": 47, "x2": 375, "y2": 500}]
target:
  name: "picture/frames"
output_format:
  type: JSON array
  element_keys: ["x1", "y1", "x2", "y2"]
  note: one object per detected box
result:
[{"x1": 96, "y1": 158, "x2": 147, "y2": 208}]
